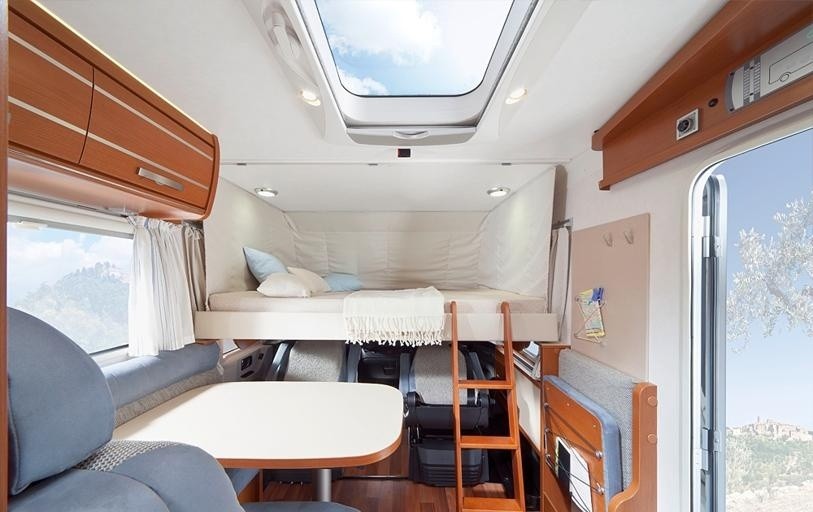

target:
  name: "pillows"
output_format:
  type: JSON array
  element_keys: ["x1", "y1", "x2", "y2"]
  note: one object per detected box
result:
[{"x1": 242, "y1": 246, "x2": 332, "y2": 297}]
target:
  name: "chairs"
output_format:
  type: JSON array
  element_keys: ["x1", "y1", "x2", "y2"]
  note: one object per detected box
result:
[
  {"x1": 539, "y1": 344, "x2": 658, "y2": 511},
  {"x1": 263, "y1": 339, "x2": 501, "y2": 432}
]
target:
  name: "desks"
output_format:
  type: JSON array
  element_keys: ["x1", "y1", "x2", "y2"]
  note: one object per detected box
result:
[{"x1": 114, "y1": 380, "x2": 404, "y2": 502}]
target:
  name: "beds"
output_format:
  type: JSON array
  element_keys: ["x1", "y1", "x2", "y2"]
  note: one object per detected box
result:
[{"x1": 193, "y1": 288, "x2": 558, "y2": 343}]
